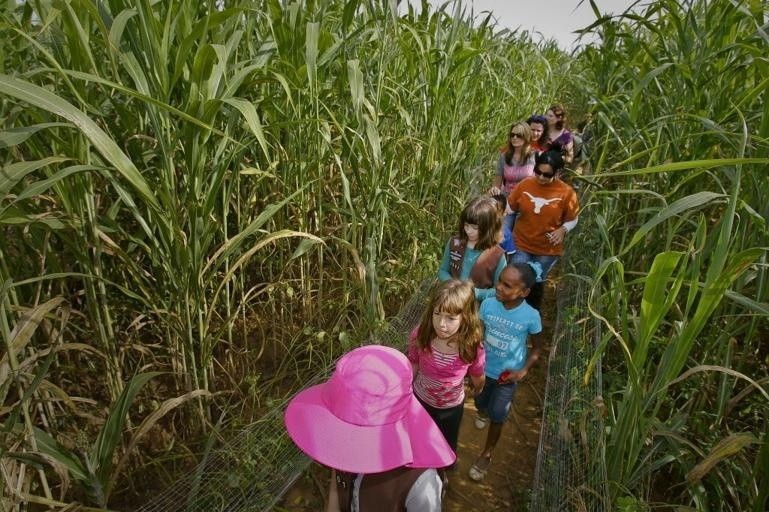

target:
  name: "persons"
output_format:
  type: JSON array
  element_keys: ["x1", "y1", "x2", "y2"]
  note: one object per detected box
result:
[
  {"x1": 469, "y1": 258, "x2": 544, "y2": 479},
  {"x1": 439, "y1": 196, "x2": 507, "y2": 300},
  {"x1": 527, "y1": 114, "x2": 548, "y2": 151},
  {"x1": 504, "y1": 150, "x2": 582, "y2": 276},
  {"x1": 287, "y1": 345, "x2": 456, "y2": 511},
  {"x1": 409, "y1": 279, "x2": 487, "y2": 500},
  {"x1": 488, "y1": 118, "x2": 536, "y2": 234},
  {"x1": 543, "y1": 106, "x2": 577, "y2": 163},
  {"x1": 490, "y1": 196, "x2": 518, "y2": 264}
]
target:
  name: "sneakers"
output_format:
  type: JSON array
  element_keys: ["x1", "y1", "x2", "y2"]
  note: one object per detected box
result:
[
  {"x1": 469, "y1": 456, "x2": 492, "y2": 481},
  {"x1": 475, "y1": 414, "x2": 487, "y2": 429}
]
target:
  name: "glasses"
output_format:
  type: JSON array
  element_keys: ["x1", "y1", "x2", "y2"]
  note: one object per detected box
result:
[
  {"x1": 510, "y1": 133, "x2": 522, "y2": 137},
  {"x1": 534, "y1": 167, "x2": 554, "y2": 178}
]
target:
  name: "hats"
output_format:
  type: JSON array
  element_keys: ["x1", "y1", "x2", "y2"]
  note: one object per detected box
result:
[{"x1": 285, "y1": 345, "x2": 456, "y2": 474}]
